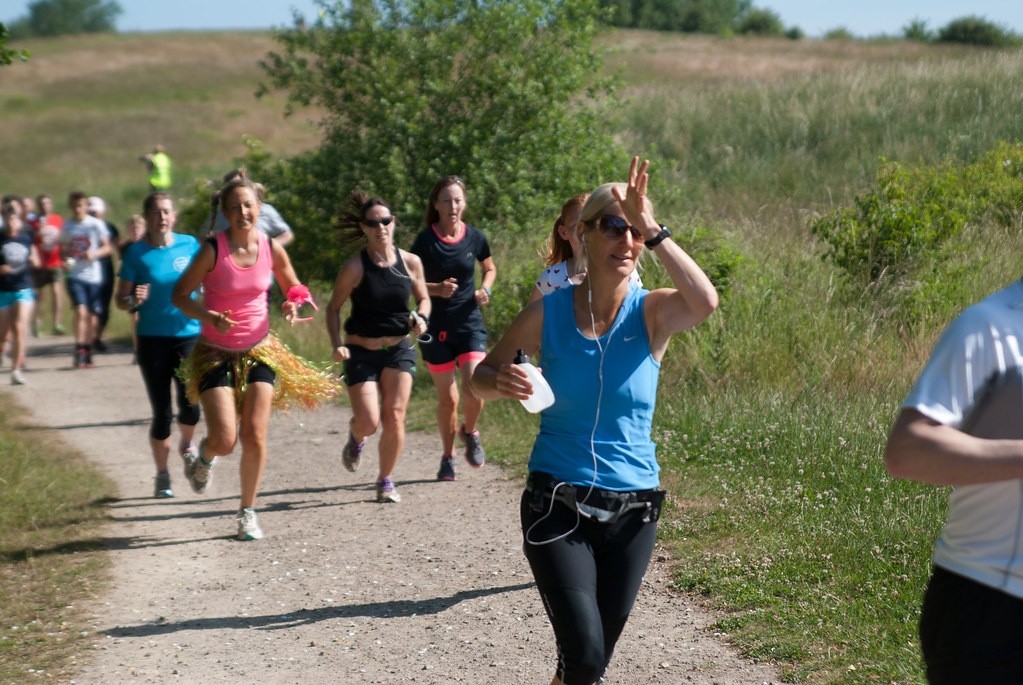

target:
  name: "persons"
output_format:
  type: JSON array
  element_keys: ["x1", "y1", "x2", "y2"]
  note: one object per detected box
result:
[
  {"x1": 324, "y1": 190, "x2": 432, "y2": 503},
  {"x1": 172, "y1": 178, "x2": 311, "y2": 542},
  {"x1": 0, "y1": 144, "x2": 297, "y2": 498},
  {"x1": 407, "y1": 175, "x2": 497, "y2": 481},
  {"x1": 468, "y1": 153, "x2": 720, "y2": 685},
  {"x1": 528, "y1": 191, "x2": 589, "y2": 307},
  {"x1": 883, "y1": 275, "x2": 1023, "y2": 684}
]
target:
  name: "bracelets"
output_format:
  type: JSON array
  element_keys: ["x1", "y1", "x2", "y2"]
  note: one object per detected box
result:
[
  {"x1": 480, "y1": 285, "x2": 493, "y2": 296},
  {"x1": 417, "y1": 313, "x2": 429, "y2": 326},
  {"x1": 127, "y1": 296, "x2": 137, "y2": 307}
]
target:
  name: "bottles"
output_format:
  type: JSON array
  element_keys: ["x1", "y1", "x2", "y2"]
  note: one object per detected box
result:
[{"x1": 513, "y1": 348, "x2": 555, "y2": 413}]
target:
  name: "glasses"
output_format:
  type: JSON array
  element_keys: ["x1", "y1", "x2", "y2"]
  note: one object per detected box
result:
[
  {"x1": 583, "y1": 213, "x2": 646, "y2": 244},
  {"x1": 361, "y1": 215, "x2": 395, "y2": 228}
]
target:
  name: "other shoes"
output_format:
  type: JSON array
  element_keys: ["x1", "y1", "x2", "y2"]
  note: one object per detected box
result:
[
  {"x1": 54, "y1": 324, "x2": 65, "y2": 335},
  {"x1": 33, "y1": 320, "x2": 41, "y2": 338},
  {"x1": 75, "y1": 346, "x2": 91, "y2": 368},
  {"x1": 11, "y1": 369, "x2": 25, "y2": 385},
  {"x1": 92, "y1": 338, "x2": 107, "y2": 351}
]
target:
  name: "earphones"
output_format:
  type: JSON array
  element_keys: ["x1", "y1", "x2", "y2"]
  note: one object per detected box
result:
[{"x1": 581, "y1": 231, "x2": 585, "y2": 241}]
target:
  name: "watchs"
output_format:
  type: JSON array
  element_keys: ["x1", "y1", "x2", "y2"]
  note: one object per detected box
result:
[{"x1": 644, "y1": 223, "x2": 672, "y2": 251}]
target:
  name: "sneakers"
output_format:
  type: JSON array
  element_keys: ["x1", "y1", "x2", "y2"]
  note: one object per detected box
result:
[
  {"x1": 178, "y1": 438, "x2": 198, "y2": 478},
  {"x1": 342, "y1": 431, "x2": 365, "y2": 472},
  {"x1": 377, "y1": 474, "x2": 400, "y2": 502},
  {"x1": 459, "y1": 423, "x2": 485, "y2": 468},
  {"x1": 236, "y1": 506, "x2": 262, "y2": 541},
  {"x1": 189, "y1": 456, "x2": 215, "y2": 493},
  {"x1": 437, "y1": 455, "x2": 456, "y2": 481},
  {"x1": 153, "y1": 470, "x2": 174, "y2": 499}
]
